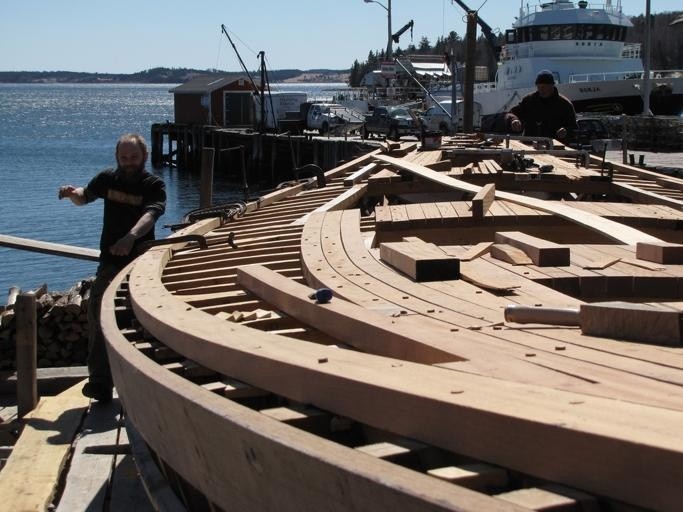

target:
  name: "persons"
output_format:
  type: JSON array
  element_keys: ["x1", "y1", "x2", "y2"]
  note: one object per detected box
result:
[
  {"x1": 503, "y1": 69, "x2": 576, "y2": 139},
  {"x1": 59, "y1": 134, "x2": 167, "y2": 401}
]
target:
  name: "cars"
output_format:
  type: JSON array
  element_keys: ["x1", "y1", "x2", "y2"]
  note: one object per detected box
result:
[{"x1": 569, "y1": 118, "x2": 610, "y2": 147}]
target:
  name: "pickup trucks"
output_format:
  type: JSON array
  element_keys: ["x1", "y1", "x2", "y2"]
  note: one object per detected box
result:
[
  {"x1": 359, "y1": 105, "x2": 426, "y2": 142},
  {"x1": 285, "y1": 103, "x2": 350, "y2": 135}
]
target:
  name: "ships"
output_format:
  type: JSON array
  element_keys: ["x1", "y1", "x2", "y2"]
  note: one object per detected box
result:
[{"x1": 426, "y1": 1, "x2": 683, "y2": 116}]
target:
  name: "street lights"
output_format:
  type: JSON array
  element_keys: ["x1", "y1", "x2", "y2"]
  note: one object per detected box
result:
[{"x1": 364, "y1": 0, "x2": 393, "y2": 61}]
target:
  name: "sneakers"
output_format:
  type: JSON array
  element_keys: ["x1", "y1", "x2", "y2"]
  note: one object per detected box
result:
[{"x1": 81, "y1": 380, "x2": 114, "y2": 406}]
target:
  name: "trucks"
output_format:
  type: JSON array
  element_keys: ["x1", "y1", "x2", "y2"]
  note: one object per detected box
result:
[{"x1": 421, "y1": 99, "x2": 482, "y2": 135}]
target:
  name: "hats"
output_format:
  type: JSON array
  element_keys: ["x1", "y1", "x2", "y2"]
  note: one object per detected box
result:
[{"x1": 535, "y1": 70, "x2": 556, "y2": 89}]
